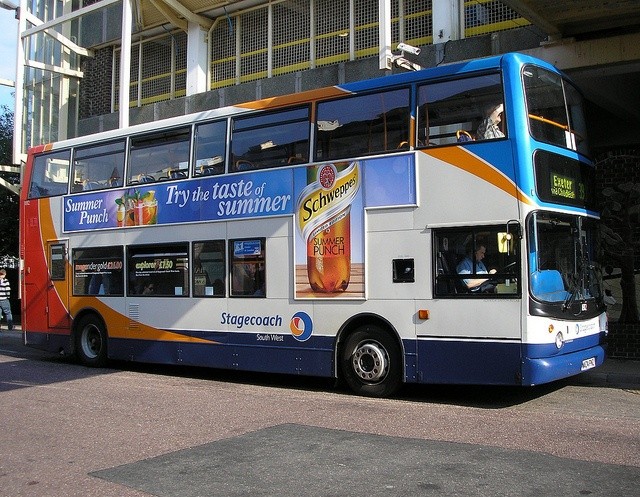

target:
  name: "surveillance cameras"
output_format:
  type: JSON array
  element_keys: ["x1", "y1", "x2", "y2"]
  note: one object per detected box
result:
[{"x1": 397, "y1": 42, "x2": 421, "y2": 56}]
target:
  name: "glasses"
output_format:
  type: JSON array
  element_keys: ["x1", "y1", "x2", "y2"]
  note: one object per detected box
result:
[{"x1": 147, "y1": 288, "x2": 156, "y2": 290}]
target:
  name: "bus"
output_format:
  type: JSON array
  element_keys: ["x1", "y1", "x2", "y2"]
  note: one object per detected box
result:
[{"x1": 18, "y1": 53, "x2": 610, "y2": 396}]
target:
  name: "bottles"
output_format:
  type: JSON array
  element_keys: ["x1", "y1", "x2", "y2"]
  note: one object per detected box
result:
[
  {"x1": 305, "y1": 161, "x2": 352, "y2": 293},
  {"x1": 116, "y1": 210, "x2": 134, "y2": 226}
]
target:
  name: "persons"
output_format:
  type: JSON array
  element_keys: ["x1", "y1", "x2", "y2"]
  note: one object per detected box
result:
[
  {"x1": 475, "y1": 103, "x2": 505, "y2": 141},
  {"x1": 142, "y1": 280, "x2": 156, "y2": 294},
  {"x1": 0, "y1": 268, "x2": 14, "y2": 330},
  {"x1": 456, "y1": 242, "x2": 497, "y2": 293}
]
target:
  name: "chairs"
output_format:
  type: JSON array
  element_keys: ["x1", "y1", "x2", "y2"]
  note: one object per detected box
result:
[
  {"x1": 236, "y1": 159, "x2": 256, "y2": 170},
  {"x1": 397, "y1": 141, "x2": 408, "y2": 149},
  {"x1": 457, "y1": 129, "x2": 473, "y2": 141},
  {"x1": 137, "y1": 173, "x2": 155, "y2": 183},
  {"x1": 288, "y1": 156, "x2": 306, "y2": 164},
  {"x1": 158, "y1": 177, "x2": 168, "y2": 180},
  {"x1": 173, "y1": 170, "x2": 186, "y2": 179},
  {"x1": 88, "y1": 181, "x2": 101, "y2": 190},
  {"x1": 201, "y1": 165, "x2": 219, "y2": 174},
  {"x1": 439, "y1": 250, "x2": 464, "y2": 294},
  {"x1": 167, "y1": 170, "x2": 174, "y2": 179},
  {"x1": 130, "y1": 181, "x2": 138, "y2": 184}
]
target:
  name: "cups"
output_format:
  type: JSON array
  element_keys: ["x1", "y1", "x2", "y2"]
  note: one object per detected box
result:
[{"x1": 134, "y1": 203, "x2": 158, "y2": 227}]
